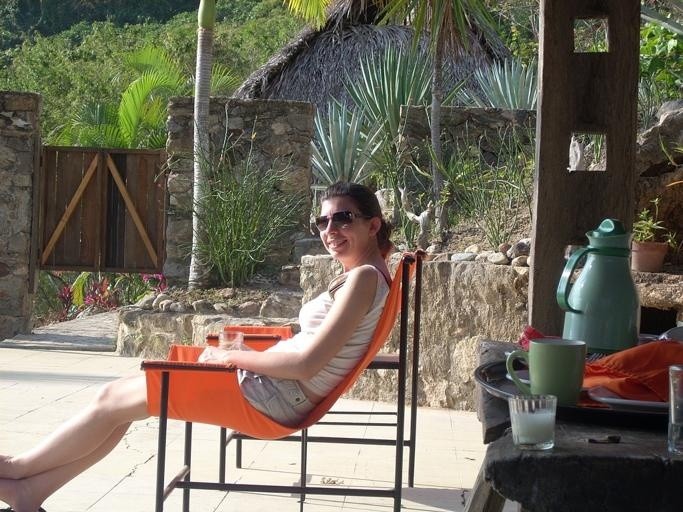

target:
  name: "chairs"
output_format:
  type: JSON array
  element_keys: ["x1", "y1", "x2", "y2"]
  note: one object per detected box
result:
[
  {"x1": 205, "y1": 249, "x2": 427, "y2": 490},
  {"x1": 140, "y1": 253, "x2": 413, "y2": 512}
]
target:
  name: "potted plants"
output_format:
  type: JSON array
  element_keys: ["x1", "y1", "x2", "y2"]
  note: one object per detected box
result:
[{"x1": 629, "y1": 199, "x2": 675, "y2": 272}]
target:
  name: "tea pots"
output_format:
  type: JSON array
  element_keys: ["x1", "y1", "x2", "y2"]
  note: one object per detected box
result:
[{"x1": 555, "y1": 217, "x2": 642, "y2": 360}]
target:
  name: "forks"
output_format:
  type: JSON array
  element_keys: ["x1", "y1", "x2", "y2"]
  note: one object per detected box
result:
[{"x1": 586, "y1": 351, "x2": 605, "y2": 362}]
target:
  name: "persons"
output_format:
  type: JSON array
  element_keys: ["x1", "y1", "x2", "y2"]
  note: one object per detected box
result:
[{"x1": 0, "y1": 183, "x2": 399, "y2": 511}]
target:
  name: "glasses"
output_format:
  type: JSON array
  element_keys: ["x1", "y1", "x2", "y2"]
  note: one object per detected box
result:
[{"x1": 316, "y1": 211, "x2": 372, "y2": 231}]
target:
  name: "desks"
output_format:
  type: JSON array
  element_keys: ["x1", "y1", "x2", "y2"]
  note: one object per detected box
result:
[{"x1": 464, "y1": 337, "x2": 682, "y2": 511}]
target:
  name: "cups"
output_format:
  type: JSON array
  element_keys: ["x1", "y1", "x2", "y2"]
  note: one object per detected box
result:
[
  {"x1": 665, "y1": 363, "x2": 682, "y2": 456},
  {"x1": 505, "y1": 336, "x2": 587, "y2": 406},
  {"x1": 217, "y1": 330, "x2": 244, "y2": 351},
  {"x1": 507, "y1": 392, "x2": 559, "y2": 450}
]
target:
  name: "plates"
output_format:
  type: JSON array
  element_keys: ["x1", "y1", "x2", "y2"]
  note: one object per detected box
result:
[
  {"x1": 506, "y1": 369, "x2": 530, "y2": 385},
  {"x1": 588, "y1": 387, "x2": 665, "y2": 409}
]
